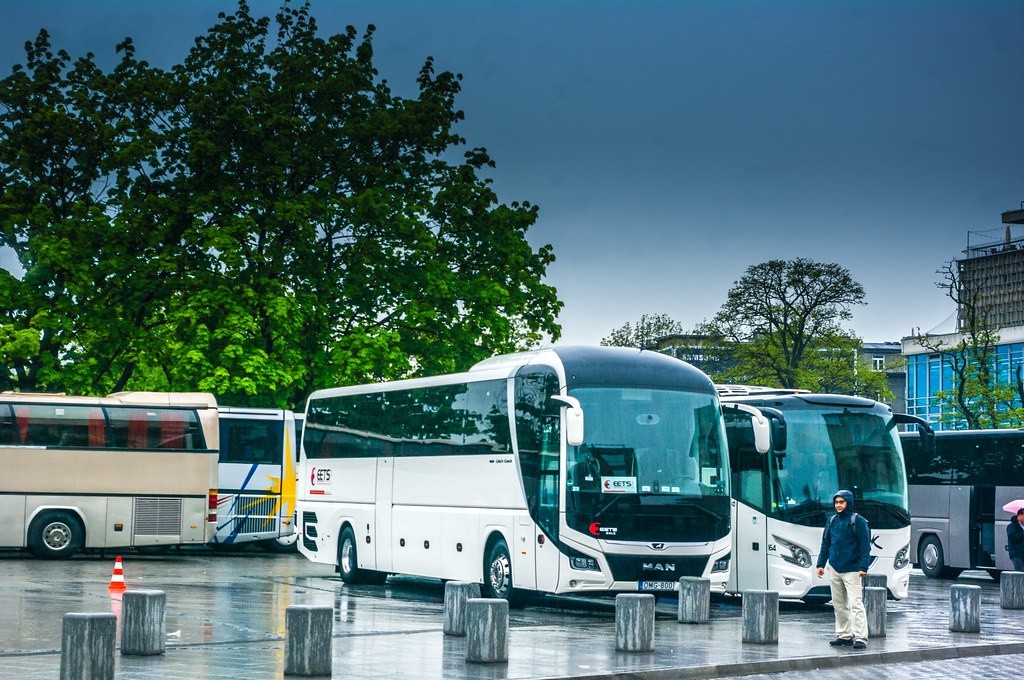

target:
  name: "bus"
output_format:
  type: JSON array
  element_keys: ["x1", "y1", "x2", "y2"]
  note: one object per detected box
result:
[
  {"x1": 294, "y1": 342, "x2": 733, "y2": 603},
  {"x1": 712, "y1": 381, "x2": 937, "y2": 614},
  {"x1": 896, "y1": 427, "x2": 1024, "y2": 581},
  {"x1": 208, "y1": 404, "x2": 305, "y2": 555},
  {"x1": 0, "y1": 390, "x2": 222, "y2": 562}
]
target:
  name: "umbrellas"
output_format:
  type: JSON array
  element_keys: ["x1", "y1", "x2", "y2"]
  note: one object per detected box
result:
[{"x1": 1002, "y1": 499, "x2": 1024, "y2": 514}]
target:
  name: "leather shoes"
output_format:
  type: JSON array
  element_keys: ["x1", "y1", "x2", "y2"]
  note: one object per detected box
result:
[
  {"x1": 830, "y1": 638, "x2": 853, "y2": 646},
  {"x1": 853, "y1": 641, "x2": 867, "y2": 650}
]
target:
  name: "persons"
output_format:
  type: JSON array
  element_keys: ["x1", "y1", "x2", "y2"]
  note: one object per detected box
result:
[
  {"x1": 816, "y1": 489, "x2": 871, "y2": 649},
  {"x1": 1006, "y1": 508, "x2": 1024, "y2": 571}
]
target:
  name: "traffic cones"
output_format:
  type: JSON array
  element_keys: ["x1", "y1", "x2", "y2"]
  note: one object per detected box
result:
[{"x1": 108, "y1": 556, "x2": 129, "y2": 590}]
